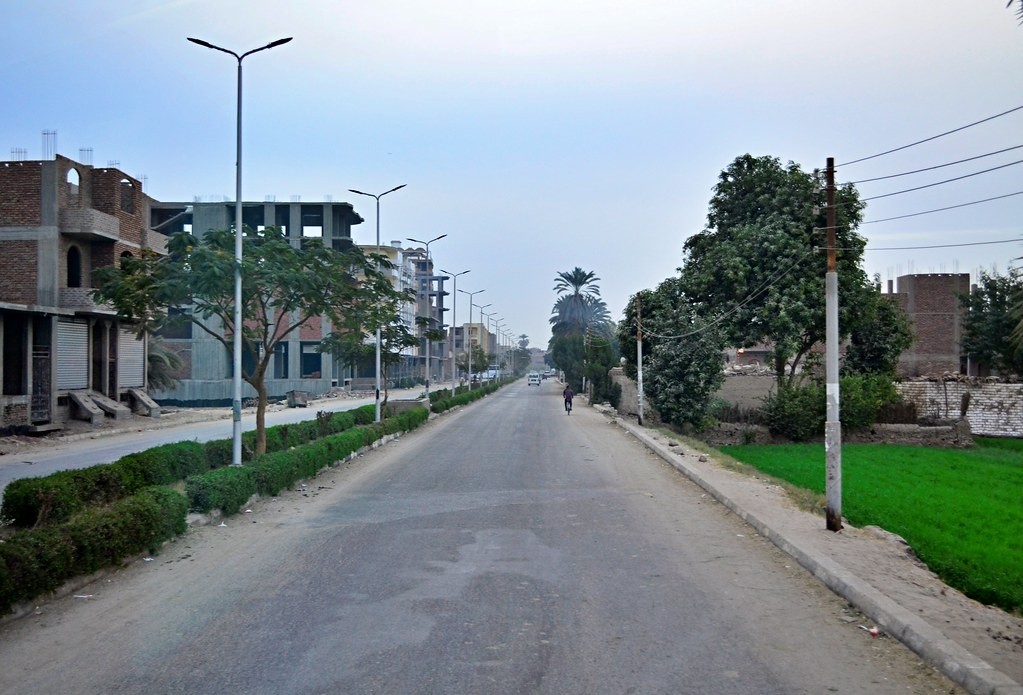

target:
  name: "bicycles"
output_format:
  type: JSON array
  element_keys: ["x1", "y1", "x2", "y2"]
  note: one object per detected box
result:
[{"x1": 564, "y1": 397, "x2": 574, "y2": 415}]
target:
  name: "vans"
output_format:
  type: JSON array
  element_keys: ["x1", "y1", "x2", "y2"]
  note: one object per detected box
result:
[
  {"x1": 544, "y1": 371, "x2": 550, "y2": 378},
  {"x1": 528, "y1": 373, "x2": 540, "y2": 386}
]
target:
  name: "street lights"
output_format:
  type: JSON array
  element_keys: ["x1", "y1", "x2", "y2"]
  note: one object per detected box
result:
[
  {"x1": 438, "y1": 268, "x2": 471, "y2": 397},
  {"x1": 457, "y1": 288, "x2": 486, "y2": 391},
  {"x1": 472, "y1": 302, "x2": 520, "y2": 388},
  {"x1": 348, "y1": 183, "x2": 406, "y2": 424},
  {"x1": 406, "y1": 234, "x2": 448, "y2": 397},
  {"x1": 186, "y1": 36, "x2": 295, "y2": 468}
]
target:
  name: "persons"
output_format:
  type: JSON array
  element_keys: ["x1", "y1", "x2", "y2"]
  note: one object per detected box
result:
[
  {"x1": 465, "y1": 372, "x2": 468, "y2": 382},
  {"x1": 563, "y1": 386, "x2": 573, "y2": 411}
]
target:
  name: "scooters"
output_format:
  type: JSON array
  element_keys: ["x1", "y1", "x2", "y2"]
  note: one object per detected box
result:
[{"x1": 542, "y1": 375, "x2": 547, "y2": 381}]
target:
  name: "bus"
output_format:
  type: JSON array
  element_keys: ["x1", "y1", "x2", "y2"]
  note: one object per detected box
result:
[{"x1": 470, "y1": 364, "x2": 500, "y2": 379}]
